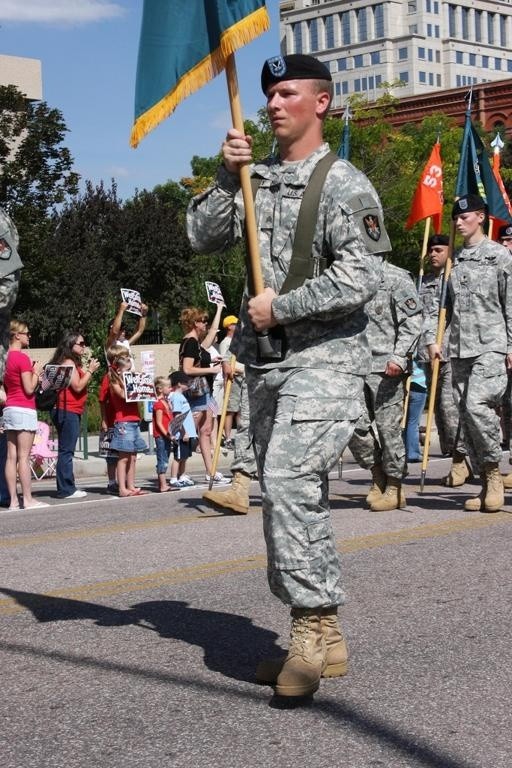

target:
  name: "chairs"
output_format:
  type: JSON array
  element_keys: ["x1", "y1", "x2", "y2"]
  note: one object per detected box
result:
[{"x1": 28, "y1": 420, "x2": 58, "y2": 482}]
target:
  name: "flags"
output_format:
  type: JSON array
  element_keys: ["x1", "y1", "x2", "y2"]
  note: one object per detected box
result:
[
  {"x1": 453, "y1": 111, "x2": 511, "y2": 226},
  {"x1": 131, "y1": 1, "x2": 270, "y2": 149},
  {"x1": 404, "y1": 143, "x2": 445, "y2": 236},
  {"x1": 487, "y1": 152, "x2": 511, "y2": 245},
  {"x1": 338, "y1": 124, "x2": 352, "y2": 163}
]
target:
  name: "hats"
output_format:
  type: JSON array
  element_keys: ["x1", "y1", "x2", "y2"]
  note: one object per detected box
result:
[
  {"x1": 427, "y1": 233, "x2": 450, "y2": 249},
  {"x1": 259, "y1": 52, "x2": 334, "y2": 96},
  {"x1": 497, "y1": 224, "x2": 512, "y2": 241},
  {"x1": 450, "y1": 192, "x2": 486, "y2": 221}
]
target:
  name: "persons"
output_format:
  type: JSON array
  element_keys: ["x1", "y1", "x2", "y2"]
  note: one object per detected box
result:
[
  {"x1": 187, "y1": 54, "x2": 397, "y2": 699},
  {"x1": 348, "y1": 193, "x2": 512, "y2": 512},
  {"x1": 0, "y1": 208, "x2": 259, "y2": 515}
]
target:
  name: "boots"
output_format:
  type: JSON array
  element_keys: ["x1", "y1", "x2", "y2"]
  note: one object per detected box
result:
[
  {"x1": 369, "y1": 478, "x2": 408, "y2": 512},
  {"x1": 444, "y1": 454, "x2": 472, "y2": 487},
  {"x1": 274, "y1": 605, "x2": 330, "y2": 700},
  {"x1": 254, "y1": 604, "x2": 349, "y2": 687},
  {"x1": 500, "y1": 470, "x2": 511, "y2": 490},
  {"x1": 483, "y1": 462, "x2": 505, "y2": 514},
  {"x1": 364, "y1": 464, "x2": 386, "y2": 507},
  {"x1": 201, "y1": 470, "x2": 252, "y2": 516},
  {"x1": 464, "y1": 471, "x2": 487, "y2": 513},
  {"x1": 440, "y1": 468, "x2": 476, "y2": 487}
]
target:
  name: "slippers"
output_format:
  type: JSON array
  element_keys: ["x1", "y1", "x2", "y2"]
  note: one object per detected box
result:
[
  {"x1": 23, "y1": 501, "x2": 51, "y2": 511},
  {"x1": 7, "y1": 498, "x2": 23, "y2": 511}
]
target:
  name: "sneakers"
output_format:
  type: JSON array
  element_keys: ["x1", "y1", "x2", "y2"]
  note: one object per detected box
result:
[
  {"x1": 63, "y1": 488, "x2": 88, "y2": 500},
  {"x1": 105, "y1": 473, "x2": 233, "y2": 499}
]
